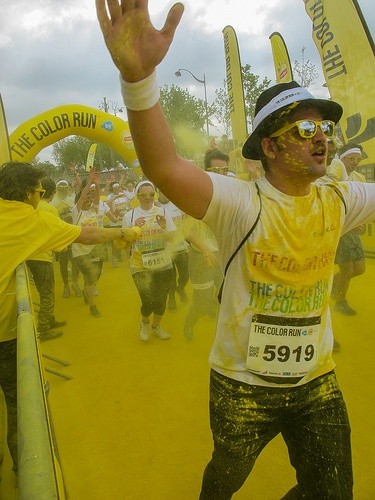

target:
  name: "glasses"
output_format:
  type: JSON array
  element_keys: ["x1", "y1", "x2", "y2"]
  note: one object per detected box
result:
[
  {"x1": 35, "y1": 188, "x2": 46, "y2": 198},
  {"x1": 343, "y1": 155, "x2": 363, "y2": 162},
  {"x1": 137, "y1": 191, "x2": 156, "y2": 198},
  {"x1": 269, "y1": 119, "x2": 336, "y2": 139},
  {"x1": 56, "y1": 186, "x2": 68, "y2": 192},
  {"x1": 54, "y1": 191, "x2": 56, "y2": 195},
  {"x1": 206, "y1": 166, "x2": 229, "y2": 173},
  {"x1": 327, "y1": 150, "x2": 337, "y2": 157}
]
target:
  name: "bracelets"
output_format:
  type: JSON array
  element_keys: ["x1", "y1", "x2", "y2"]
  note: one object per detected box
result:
[
  {"x1": 120, "y1": 68, "x2": 160, "y2": 111},
  {"x1": 120, "y1": 228, "x2": 125, "y2": 240}
]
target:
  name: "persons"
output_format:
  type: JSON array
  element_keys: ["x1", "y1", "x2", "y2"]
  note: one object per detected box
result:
[
  {"x1": 0, "y1": 145, "x2": 375, "y2": 477},
  {"x1": 96, "y1": 0, "x2": 375, "y2": 500}
]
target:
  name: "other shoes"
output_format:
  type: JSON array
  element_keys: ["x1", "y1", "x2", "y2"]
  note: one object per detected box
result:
[
  {"x1": 37, "y1": 330, "x2": 63, "y2": 342},
  {"x1": 50, "y1": 319, "x2": 67, "y2": 329},
  {"x1": 334, "y1": 299, "x2": 357, "y2": 316},
  {"x1": 55, "y1": 252, "x2": 123, "y2": 317},
  {"x1": 167, "y1": 294, "x2": 177, "y2": 310},
  {"x1": 332, "y1": 338, "x2": 341, "y2": 351},
  {"x1": 139, "y1": 322, "x2": 150, "y2": 342},
  {"x1": 176, "y1": 284, "x2": 189, "y2": 303},
  {"x1": 151, "y1": 325, "x2": 172, "y2": 340}
]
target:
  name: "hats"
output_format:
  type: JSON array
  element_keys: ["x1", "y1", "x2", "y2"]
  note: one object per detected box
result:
[
  {"x1": 339, "y1": 142, "x2": 364, "y2": 160},
  {"x1": 242, "y1": 81, "x2": 343, "y2": 160},
  {"x1": 55, "y1": 178, "x2": 68, "y2": 189}
]
